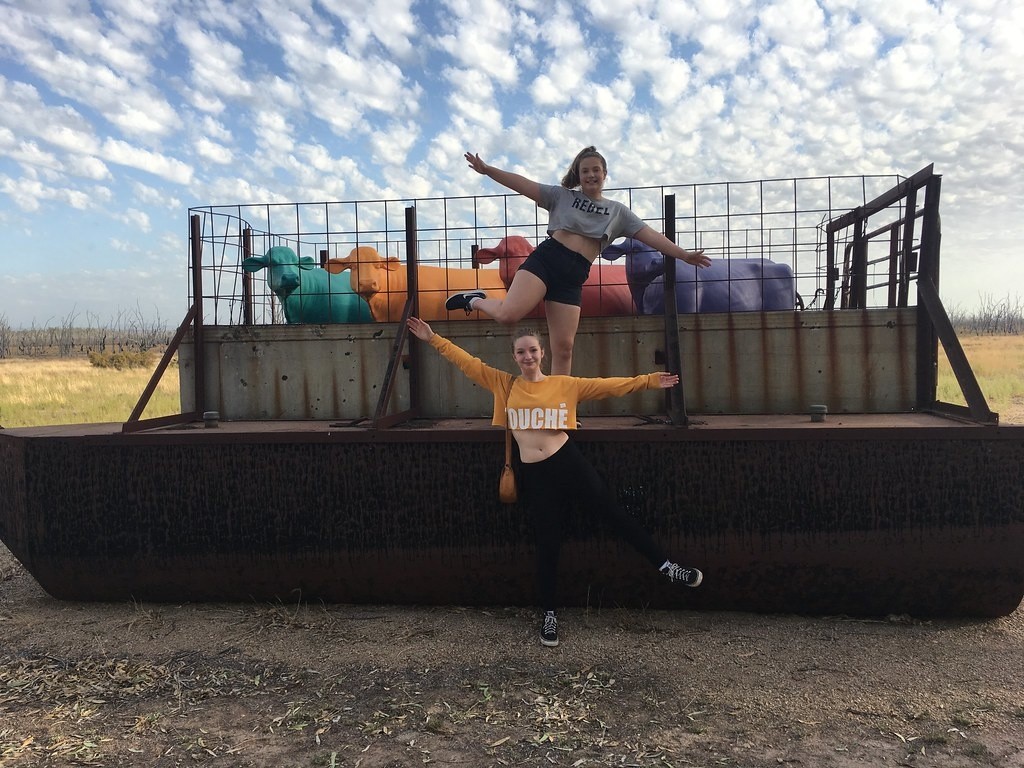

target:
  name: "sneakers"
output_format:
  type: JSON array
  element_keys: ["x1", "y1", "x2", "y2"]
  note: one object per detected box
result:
[
  {"x1": 446, "y1": 290, "x2": 487, "y2": 316},
  {"x1": 539, "y1": 610, "x2": 559, "y2": 646},
  {"x1": 658, "y1": 558, "x2": 703, "y2": 587},
  {"x1": 577, "y1": 418, "x2": 582, "y2": 427}
]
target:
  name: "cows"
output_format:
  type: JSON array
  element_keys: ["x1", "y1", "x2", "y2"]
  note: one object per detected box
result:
[{"x1": 241, "y1": 234, "x2": 796, "y2": 327}]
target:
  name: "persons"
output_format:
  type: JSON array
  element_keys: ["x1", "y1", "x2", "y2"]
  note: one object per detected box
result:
[
  {"x1": 443, "y1": 145, "x2": 712, "y2": 426},
  {"x1": 407, "y1": 316, "x2": 702, "y2": 648}
]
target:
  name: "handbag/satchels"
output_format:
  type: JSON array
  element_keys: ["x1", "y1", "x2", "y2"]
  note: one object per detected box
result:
[{"x1": 499, "y1": 464, "x2": 517, "y2": 504}]
